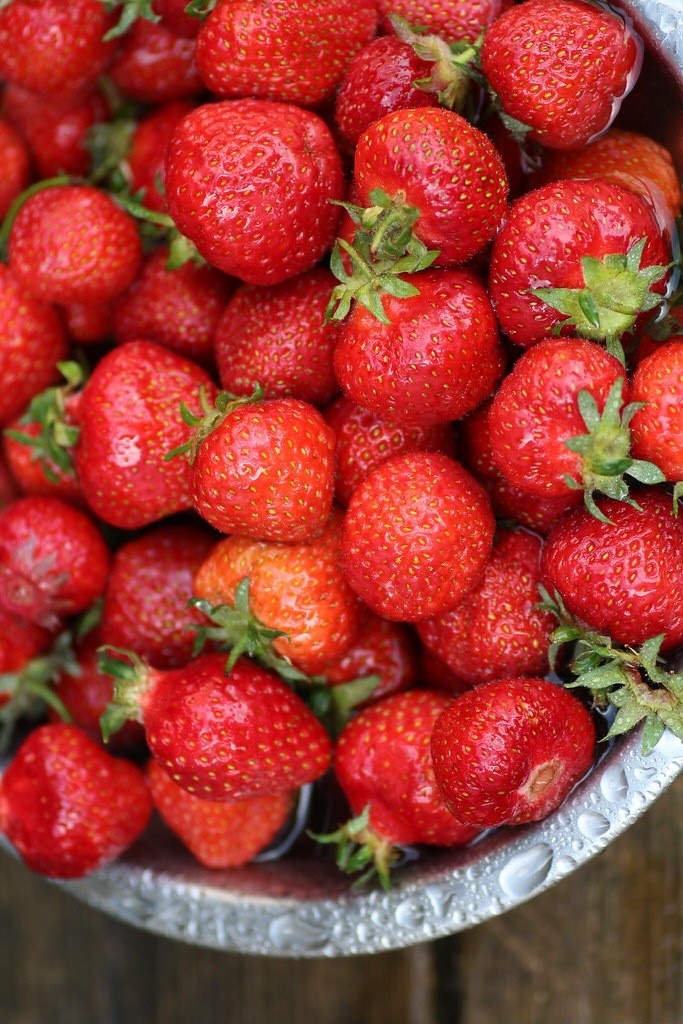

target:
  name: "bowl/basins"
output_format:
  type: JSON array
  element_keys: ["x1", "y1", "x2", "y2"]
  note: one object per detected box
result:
[{"x1": 0, "y1": 0, "x2": 683, "y2": 960}]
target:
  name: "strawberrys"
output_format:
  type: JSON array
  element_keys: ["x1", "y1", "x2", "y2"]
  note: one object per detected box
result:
[{"x1": 0, "y1": 0, "x2": 683, "y2": 879}]
